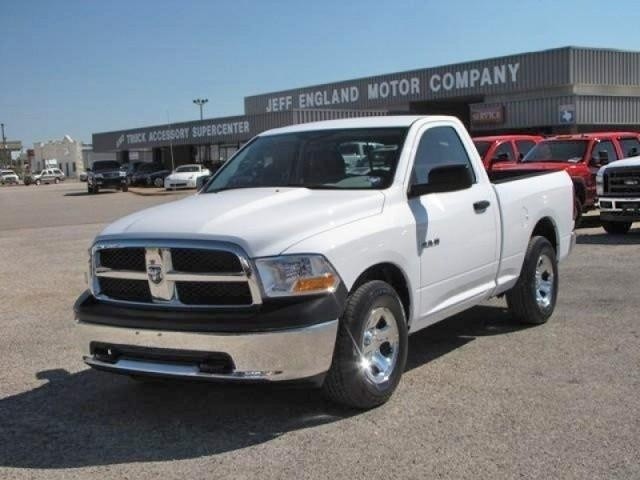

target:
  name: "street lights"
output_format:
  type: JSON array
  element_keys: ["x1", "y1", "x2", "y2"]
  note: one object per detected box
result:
[
  {"x1": 0, "y1": 122, "x2": 10, "y2": 165},
  {"x1": 193, "y1": 98, "x2": 209, "y2": 120}
]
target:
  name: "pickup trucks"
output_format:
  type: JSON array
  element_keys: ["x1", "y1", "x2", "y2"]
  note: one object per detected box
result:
[{"x1": 71, "y1": 113, "x2": 582, "y2": 419}]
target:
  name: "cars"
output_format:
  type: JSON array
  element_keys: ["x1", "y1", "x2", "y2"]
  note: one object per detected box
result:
[
  {"x1": 120, "y1": 158, "x2": 213, "y2": 192},
  {"x1": 80, "y1": 170, "x2": 88, "y2": 181},
  {"x1": 22, "y1": 167, "x2": 67, "y2": 186}
]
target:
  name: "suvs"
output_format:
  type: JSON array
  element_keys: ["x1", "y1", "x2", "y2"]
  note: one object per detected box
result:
[
  {"x1": 0, "y1": 168, "x2": 20, "y2": 185},
  {"x1": 84, "y1": 159, "x2": 130, "y2": 195}
]
